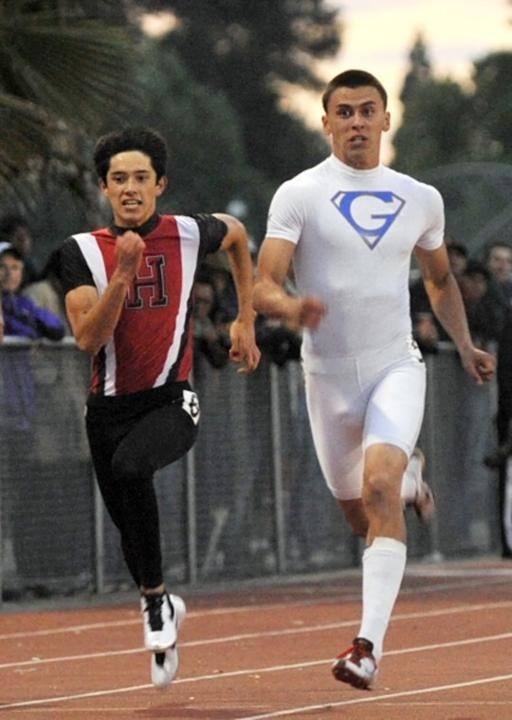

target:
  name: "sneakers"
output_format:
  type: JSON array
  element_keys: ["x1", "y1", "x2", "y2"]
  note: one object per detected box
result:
[
  {"x1": 407, "y1": 448, "x2": 432, "y2": 523},
  {"x1": 332, "y1": 636, "x2": 377, "y2": 691},
  {"x1": 140, "y1": 591, "x2": 177, "y2": 652},
  {"x1": 146, "y1": 597, "x2": 184, "y2": 685}
]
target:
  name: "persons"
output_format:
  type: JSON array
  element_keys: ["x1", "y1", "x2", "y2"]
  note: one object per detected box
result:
[
  {"x1": 251, "y1": 69, "x2": 498, "y2": 694},
  {"x1": 401, "y1": 236, "x2": 509, "y2": 564},
  {"x1": 55, "y1": 127, "x2": 263, "y2": 689},
  {"x1": 1, "y1": 238, "x2": 139, "y2": 600},
  {"x1": 153, "y1": 263, "x2": 358, "y2": 583}
]
